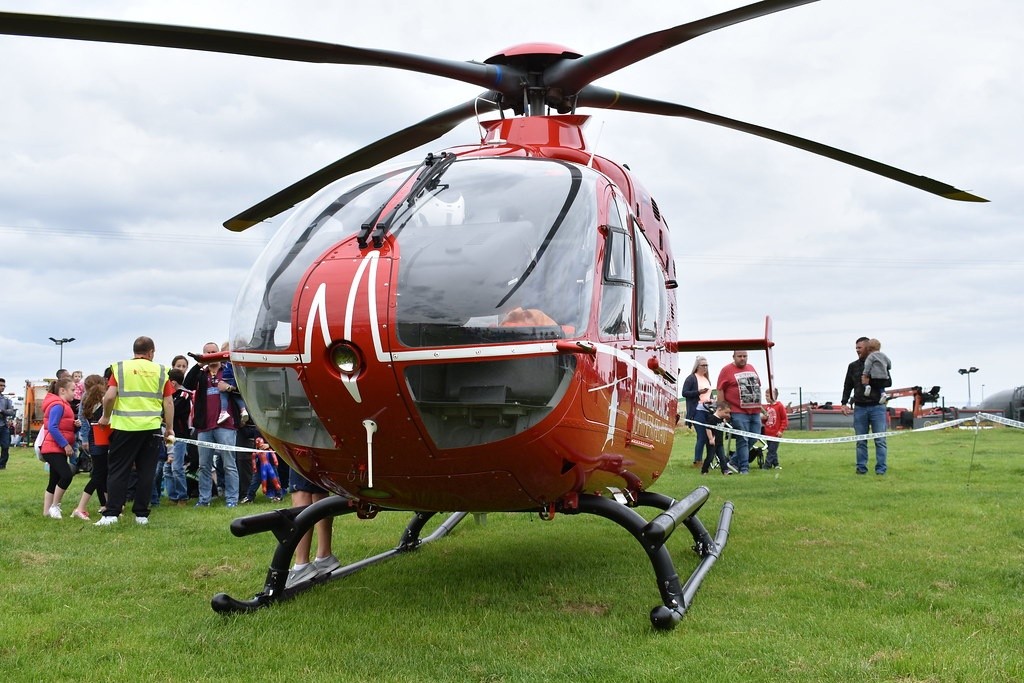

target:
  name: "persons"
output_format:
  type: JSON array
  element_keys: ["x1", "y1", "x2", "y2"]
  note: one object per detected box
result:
[
  {"x1": 0, "y1": 336, "x2": 289, "y2": 527},
  {"x1": 841, "y1": 337, "x2": 893, "y2": 475},
  {"x1": 285, "y1": 466, "x2": 342, "y2": 589},
  {"x1": 681, "y1": 349, "x2": 788, "y2": 475},
  {"x1": 862, "y1": 339, "x2": 891, "y2": 405}
]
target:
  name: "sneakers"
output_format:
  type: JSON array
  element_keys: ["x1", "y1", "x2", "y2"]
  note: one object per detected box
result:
[
  {"x1": 240, "y1": 409, "x2": 249, "y2": 422},
  {"x1": 43, "y1": 511, "x2": 50, "y2": 517},
  {"x1": 284, "y1": 562, "x2": 319, "y2": 589},
  {"x1": 312, "y1": 554, "x2": 341, "y2": 580},
  {"x1": 217, "y1": 412, "x2": 230, "y2": 424},
  {"x1": 49, "y1": 503, "x2": 63, "y2": 519}
]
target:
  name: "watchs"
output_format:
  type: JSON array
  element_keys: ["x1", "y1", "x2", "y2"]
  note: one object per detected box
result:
[{"x1": 226, "y1": 384, "x2": 232, "y2": 392}]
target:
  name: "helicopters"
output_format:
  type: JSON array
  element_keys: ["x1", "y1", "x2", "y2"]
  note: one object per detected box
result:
[{"x1": 0, "y1": 0, "x2": 991, "y2": 633}]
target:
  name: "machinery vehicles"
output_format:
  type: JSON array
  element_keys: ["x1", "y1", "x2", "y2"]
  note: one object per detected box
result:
[{"x1": 885, "y1": 385, "x2": 955, "y2": 430}]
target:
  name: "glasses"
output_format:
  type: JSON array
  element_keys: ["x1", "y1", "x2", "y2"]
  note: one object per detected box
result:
[{"x1": 699, "y1": 364, "x2": 708, "y2": 367}]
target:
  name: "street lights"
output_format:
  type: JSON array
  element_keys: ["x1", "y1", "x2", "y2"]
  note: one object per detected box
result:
[
  {"x1": 958, "y1": 366, "x2": 980, "y2": 408},
  {"x1": 48, "y1": 337, "x2": 76, "y2": 370}
]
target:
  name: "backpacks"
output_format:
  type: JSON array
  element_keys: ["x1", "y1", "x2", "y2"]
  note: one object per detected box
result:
[{"x1": 34, "y1": 423, "x2": 50, "y2": 463}]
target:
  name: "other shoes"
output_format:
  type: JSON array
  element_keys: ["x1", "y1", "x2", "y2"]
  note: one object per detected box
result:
[
  {"x1": 693, "y1": 461, "x2": 704, "y2": 468},
  {"x1": 864, "y1": 385, "x2": 871, "y2": 397},
  {"x1": 762, "y1": 467, "x2": 772, "y2": 470},
  {"x1": 879, "y1": 392, "x2": 887, "y2": 404},
  {"x1": 70, "y1": 509, "x2": 91, "y2": 521},
  {"x1": 702, "y1": 470, "x2": 708, "y2": 475},
  {"x1": 136, "y1": 516, "x2": 148, "y2": 524},
  {"x1": 98, "y1": 505, "x2": 107, "y2": 513},
  {"x1": 93, "y1": 516, "x2": 117, "y2": 526},
  {"x1": 150, "y1": 486, "x2": 287, "y2": 512},
  {"x1": 726, "y1": 460, "x2": 738, "y2": 474},
  {"x1": 740, "y1": 470, "x2": 748, "y2": 474},
  {"x1": 723, "y1": 470, "x2": 733, "y2": 476},
  {"x1": 775, "y1": 466, "x2": 782, "y2": 470}
]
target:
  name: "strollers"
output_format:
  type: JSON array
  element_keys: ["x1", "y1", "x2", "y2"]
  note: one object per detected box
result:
[{"x1": 702, "y1": 400, "x2": 768, "y2": 474}]
